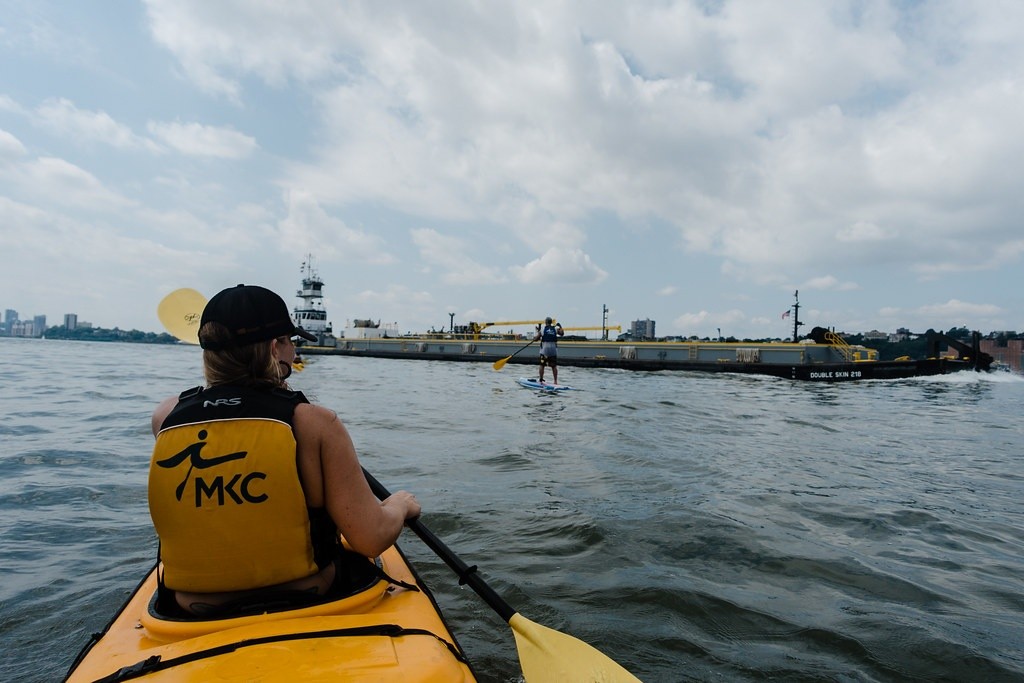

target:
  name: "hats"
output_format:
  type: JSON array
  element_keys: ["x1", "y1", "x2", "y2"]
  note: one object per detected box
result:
[{"x1": 198, "y1": 284, "x2": 318, "y2": 348}]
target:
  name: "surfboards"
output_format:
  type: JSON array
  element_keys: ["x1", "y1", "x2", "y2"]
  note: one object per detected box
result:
[{"x1": 518, "y1": 377, "x2": 585, "y2": 392}]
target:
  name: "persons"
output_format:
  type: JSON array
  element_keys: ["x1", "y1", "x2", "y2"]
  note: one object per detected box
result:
[
  {"x1": 293, "y1": 351, "x2": 302, "y2": 363},
  {"x1": 147, "y1": 284, "x2": 421, "y2": 610},
  {"x1": 534, "y1": 317, "x2": 565, "y2": 386}
]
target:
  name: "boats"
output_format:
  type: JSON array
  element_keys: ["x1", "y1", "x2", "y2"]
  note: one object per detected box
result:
[
  {"x1": 514, "y1": 376, "x2": 569, "y2": 395},
  {"x1": 291, "y1": 252, "x2": 881, "y2": 374}
]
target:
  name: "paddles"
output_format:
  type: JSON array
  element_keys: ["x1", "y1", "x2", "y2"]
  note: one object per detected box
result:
[
  {"x1": 156, "y1": 285, "x2": 643, "y2": 683},
  {"x1": 493, "y1": 324, "x2": 557, "y2": 370}
]
